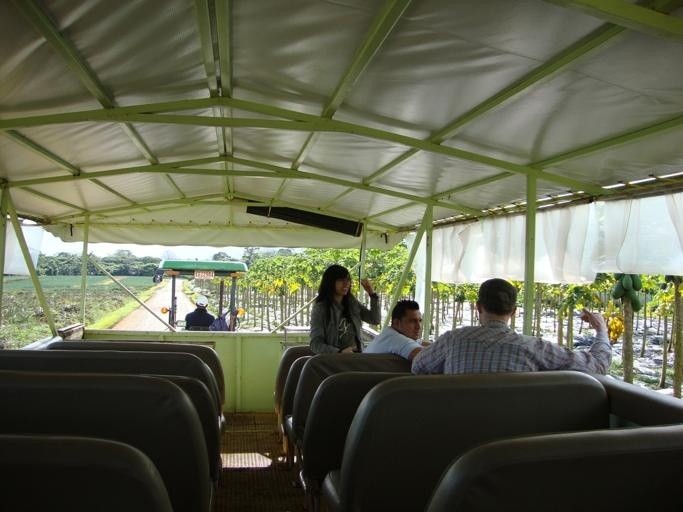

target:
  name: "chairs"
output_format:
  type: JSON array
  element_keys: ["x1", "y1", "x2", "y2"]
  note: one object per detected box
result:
[{"x1": 188, "y1": 325, "x2": 210, "y2": 331}]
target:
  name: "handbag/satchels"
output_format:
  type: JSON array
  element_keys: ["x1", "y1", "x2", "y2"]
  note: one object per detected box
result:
[{"x1": 209, "y1": 316, "x2": 230, "y2": 331}]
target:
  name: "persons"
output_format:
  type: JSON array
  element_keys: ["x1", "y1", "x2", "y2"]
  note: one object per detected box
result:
[
  {"x1": 309, "y1": 264, "x2": 381, "y2": 355},
  {"x1": 411, "y1": 277, "x2": 612, "y2": 376},
  {"x1": 360, "y1": 299, "x2": 433, "y2": 362},
  {"x1": 185, "y1": 295, "x2": 214, "y2": 330}
]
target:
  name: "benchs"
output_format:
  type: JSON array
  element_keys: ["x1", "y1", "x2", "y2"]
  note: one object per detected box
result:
[{"x1": 0, "y1": 339, "x2": 226, "y2": 512}]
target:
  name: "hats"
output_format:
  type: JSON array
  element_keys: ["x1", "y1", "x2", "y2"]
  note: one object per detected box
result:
[
  {"x1": 196, "y1": 296, "x2": 208, "y2": 307},
  {"x1": 478, "y1": 279, "x2": 517, "y2": 304}
]
